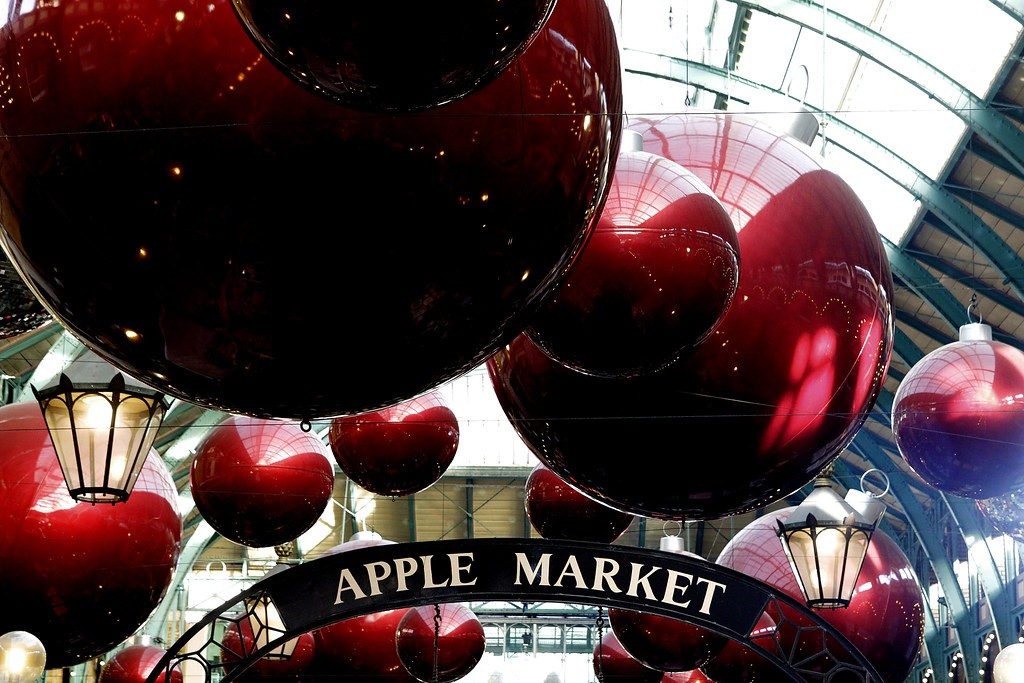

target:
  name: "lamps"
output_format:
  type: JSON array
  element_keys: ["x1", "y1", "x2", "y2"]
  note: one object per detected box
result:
[
  {"x1": 30, "y1": 349, "x2": 171, "y2": 506},
  {"x1": 775, "y1": 462, "x2": 879, "y2": 607}
]
